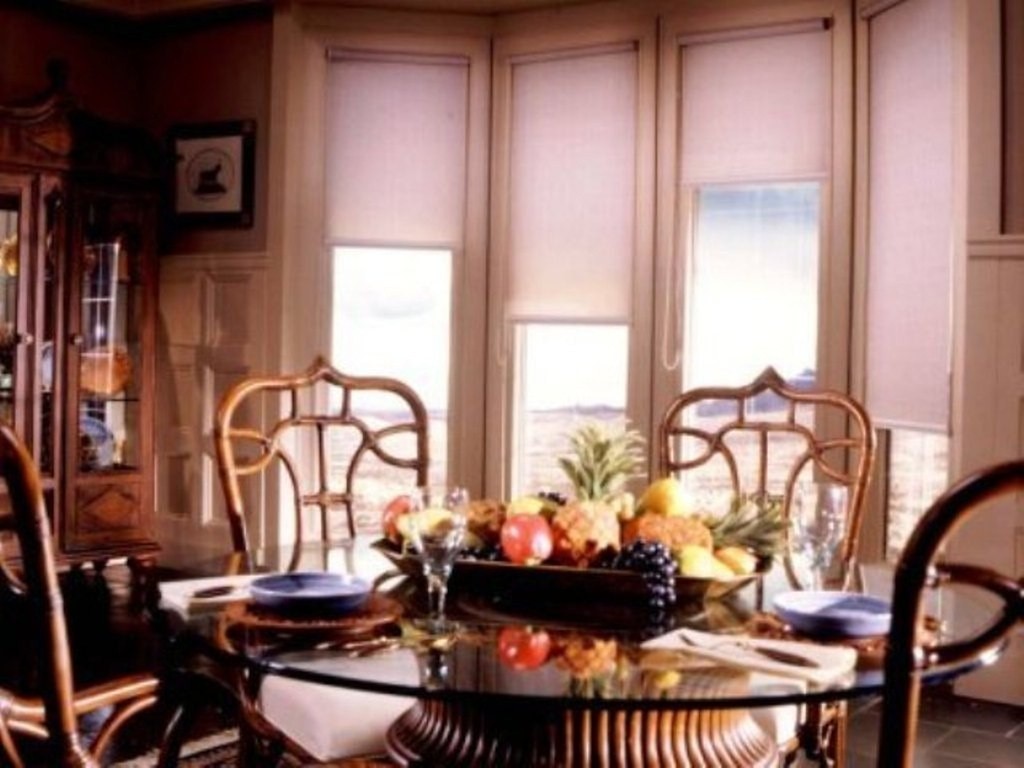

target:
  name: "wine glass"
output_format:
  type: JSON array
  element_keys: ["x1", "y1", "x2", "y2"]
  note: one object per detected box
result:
[
  {"x1": 411, "y1": 486, "x2": 470, "y2": 635},
  {"x1": 792, "y1": 483, "x2": 847, "y2": 591}
]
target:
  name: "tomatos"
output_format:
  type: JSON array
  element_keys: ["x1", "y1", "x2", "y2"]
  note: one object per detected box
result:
[{"x1": 381, "y1": 494, "x2": 423, "y2": 546}]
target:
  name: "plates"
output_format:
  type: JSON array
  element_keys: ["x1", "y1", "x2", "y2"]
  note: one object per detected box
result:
[
  {"x1": 774, "y1": 592, "x2": 890, "y2": 637},
  {"x1": 640, "y1": 626, "x2": 858, "y2": 684}
]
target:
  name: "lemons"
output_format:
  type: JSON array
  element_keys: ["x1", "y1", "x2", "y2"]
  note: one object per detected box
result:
[{"x1": 673, "y1": 546, "x2": 757, "y2": 576}]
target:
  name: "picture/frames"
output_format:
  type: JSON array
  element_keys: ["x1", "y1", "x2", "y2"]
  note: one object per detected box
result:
[
  {"x1": 167, "y1": 120, "x2": 256, "y2": 229},
  {"x1": 1, "y1": 82, "x2": 170, "y2": 575}
]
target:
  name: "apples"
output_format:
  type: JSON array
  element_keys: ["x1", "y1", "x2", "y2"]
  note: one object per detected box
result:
[
  {"x1": 497, "y1": 621, "x2": 551, "y2": 672},
  {"x1": 500, "y1": 513, "x2": 551, "y2": 564}
]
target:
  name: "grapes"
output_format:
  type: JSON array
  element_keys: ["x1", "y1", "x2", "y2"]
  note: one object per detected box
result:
[{"x1": 611, "y1": 537, "x2": 678, "y2": 626}]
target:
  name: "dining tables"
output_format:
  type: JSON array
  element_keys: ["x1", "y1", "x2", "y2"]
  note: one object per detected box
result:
[{"x1": 180, "y1": 534, "x2": 1012, "y2": 768}]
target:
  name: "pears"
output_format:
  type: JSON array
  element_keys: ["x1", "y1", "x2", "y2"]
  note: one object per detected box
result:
[{"x1": 634, "y1": 471, "x2": 688, "y2": 519}]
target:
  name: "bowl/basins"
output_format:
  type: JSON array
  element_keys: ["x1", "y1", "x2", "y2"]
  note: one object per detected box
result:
[
  {"x1": 253, "y1": 573, "x2": 372, "y2": 621},
  {"x1": 369, "y1": 538, "x2": 762, "y2": 639}
]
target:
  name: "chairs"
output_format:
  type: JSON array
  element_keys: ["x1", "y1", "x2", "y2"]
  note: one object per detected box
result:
[
  {"x1": 876, "y1": 461, "x2": 1023, "y2": 767},
  {"x1": 656, "y1": 366, "x2": 877, "y2": 599},
  {"x1": 0, "y1": 430, "x2": 257, "y2": 766},
  {"x1": 227, "y1": 353, "x2": 432, "y2": 768}
]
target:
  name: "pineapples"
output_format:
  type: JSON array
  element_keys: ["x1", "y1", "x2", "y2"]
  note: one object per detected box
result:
[
  {"x1": 558, "y1": 631, "x2": 618, "y2": 702},
  {"x1": 550, "y1": 425, "x2": 647, "y2": 563},
  {"x1": 624, "y1": 490, "x2": 791, "y2": 559},
  {"x1": 461, "y1": 499, "x2": 507, "y2": 544}
]
